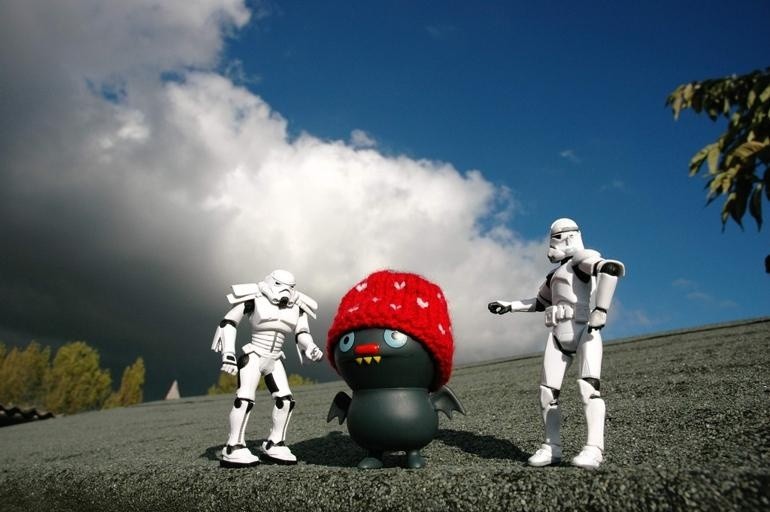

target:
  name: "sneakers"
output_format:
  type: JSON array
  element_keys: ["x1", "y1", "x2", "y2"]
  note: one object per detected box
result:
[
  {"x1": 261, "y1": 440, "x2": 297, "y2": 463},
  {"x1": 222, "y1": 445, "x2": 259, "y2": 464},
  {"x1": 571, "y1": 445, "x2": 603, "y2": 467},
  {"x1": 529, "y1": 443, "x2": 563, "y2": 465}
]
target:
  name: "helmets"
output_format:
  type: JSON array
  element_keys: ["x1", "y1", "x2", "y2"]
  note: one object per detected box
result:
[
  {"x1": 547, "y1": 218, "x2": 585, "y2": 263},
  {"x1": 259, "y1": 268, "x2": 300, "y2": 306}
]
